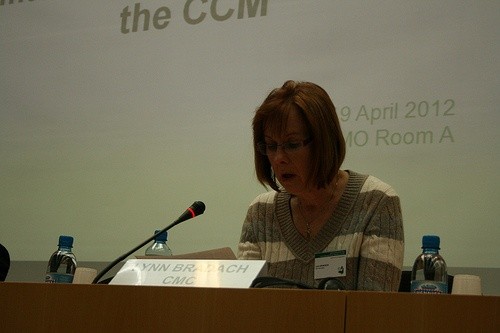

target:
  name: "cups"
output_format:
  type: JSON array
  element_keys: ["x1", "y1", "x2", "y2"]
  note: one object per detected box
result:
[
  {"x1": 73, "y1": 268, "x2": 97, "y2": 284},
  {"x1": 452, "y1": 275, "x2": 481, "y2": 295}
]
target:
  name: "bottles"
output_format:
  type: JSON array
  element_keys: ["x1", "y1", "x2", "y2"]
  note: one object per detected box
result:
[
  {"x1": 45, "y1": 235, "x2": 77, "y2": 284},
  {"x1": 145, "y1": 230, "x2": 172, "y2": 256},
  {"x1": 411, "y1": 235, "x2": 448, "y2": 293}
]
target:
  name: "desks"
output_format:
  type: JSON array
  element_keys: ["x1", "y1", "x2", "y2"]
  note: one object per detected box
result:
[{"x1": 0, "y1": 281, "x2": 500, "y2": 333}]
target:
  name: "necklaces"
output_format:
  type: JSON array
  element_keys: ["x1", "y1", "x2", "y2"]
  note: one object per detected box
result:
[{"x1": 294, "y1": 173, "x2": 340, "y2": 241}]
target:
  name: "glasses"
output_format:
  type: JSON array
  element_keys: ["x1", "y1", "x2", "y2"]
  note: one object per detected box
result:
[{"x1": 261, "y1": 135, "x2": 314, "y2": 156}]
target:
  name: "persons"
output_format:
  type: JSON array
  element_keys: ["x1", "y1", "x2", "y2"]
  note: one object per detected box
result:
[{"x1": 237, "y1": 80, "x2": 405, "y2": 292}]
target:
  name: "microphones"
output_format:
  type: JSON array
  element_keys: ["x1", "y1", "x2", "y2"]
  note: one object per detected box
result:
[{"x1": 92, "y1": 200, "x2": 206, "y2": 285}]
